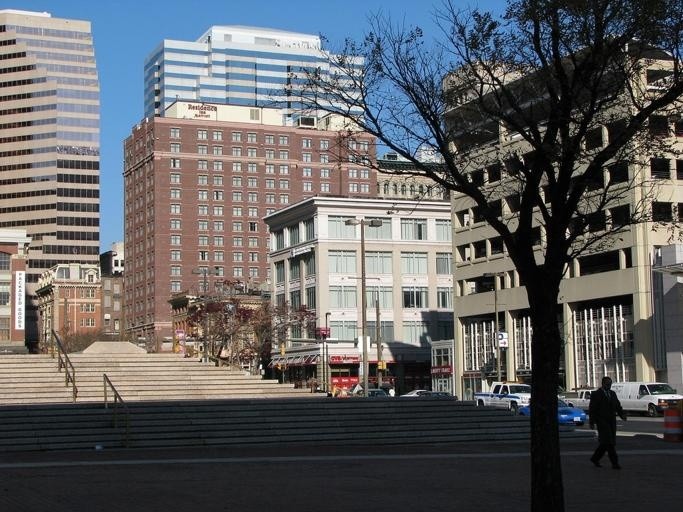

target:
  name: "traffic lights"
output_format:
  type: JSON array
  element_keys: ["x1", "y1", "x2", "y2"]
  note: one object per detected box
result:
[
  {"x1": 275, "y1": 363, "x2": 281, "y2": 371},
  {"x1": 284, "y1": 362, "x2": 289, "y2": 370}
]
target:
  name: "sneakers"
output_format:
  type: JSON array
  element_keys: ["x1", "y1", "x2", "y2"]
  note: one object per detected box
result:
[
  {"x1": 613, "y1": 465, "x2": 622, "y2": 469},
  {"x1": 591, "y1": 459, "x2": 601, "y2": 467}
]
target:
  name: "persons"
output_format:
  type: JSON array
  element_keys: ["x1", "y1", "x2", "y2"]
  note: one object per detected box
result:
[
  {"x1": 313, "y1": 376, "x2": 318, "y2": 390},
  {"x1": 333, "y1": 384, "x2": 337, "y2": 398},
  {"x1": 343, "y1": 384, "x2": 348, "y2": 394},
  {"x1": 588, "y1": 377, "x2": 626, "y2": 469},
  {"x1": 388, "y1": 386, "x2": 395, "y2": 398}
]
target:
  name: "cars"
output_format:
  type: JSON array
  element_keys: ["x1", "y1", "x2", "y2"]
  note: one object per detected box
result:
[
  {"x1": 517, "y1": 399, "x2": 586, "y2": 426},
  {"x1": 355, "y1": 388, "x2": 457, "y2": 401}
]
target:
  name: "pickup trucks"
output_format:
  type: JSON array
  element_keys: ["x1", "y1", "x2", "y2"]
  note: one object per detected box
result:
[
  {"x1": 473, "y1": 382, "x2": 531, "y2": 416},
  {"x1": 560, "y1": 389, "x2": 597, "y2": 411}
]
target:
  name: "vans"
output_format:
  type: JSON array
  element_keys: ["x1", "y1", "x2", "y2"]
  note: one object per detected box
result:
[{"x1": 610, "y1": 382, "x2": 683, "y2": 417}]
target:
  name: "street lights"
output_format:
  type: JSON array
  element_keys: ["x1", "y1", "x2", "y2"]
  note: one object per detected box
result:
[
  {"x1": 344, "y1": 219, "x2": 382, "y2": 397},
  {"x1": 483, "y1": 272, "x2": 507, "y2": 382},
  {"x1": 191, "y1": 269, "x2": 219, "y2": 358},
  {"x1": 325, "y1": 312, "x2": 330, "y2": 393}
]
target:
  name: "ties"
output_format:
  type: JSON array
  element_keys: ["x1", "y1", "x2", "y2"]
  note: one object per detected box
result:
[{"x1": 606, "y1": 392, "x2": 609, "y2": 398}]
